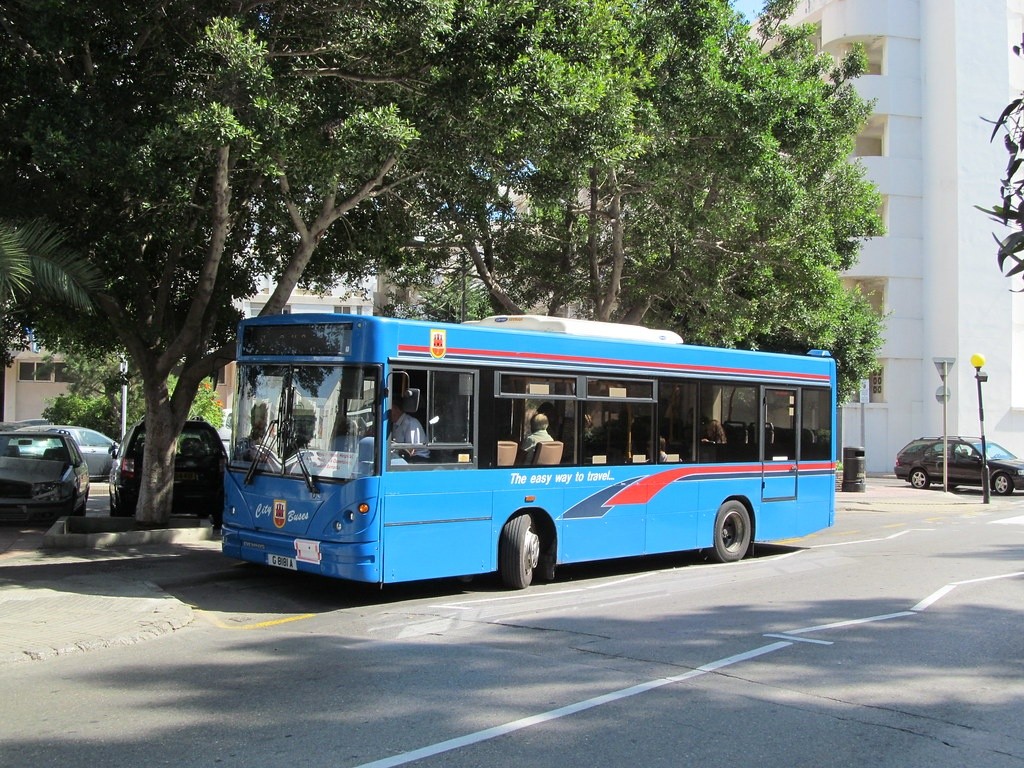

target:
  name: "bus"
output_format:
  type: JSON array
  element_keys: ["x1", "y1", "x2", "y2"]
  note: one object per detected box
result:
[{"x1": 225, "y1": 312, "x2": 841, "y2": 586}]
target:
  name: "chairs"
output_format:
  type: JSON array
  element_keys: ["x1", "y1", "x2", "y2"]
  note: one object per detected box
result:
[
  {"x1": 180, "y1": 438, "x2": 202, "y2": 455},
  {"x1": 3, "y1": 445, "x2": 20, "y2": 457},
  {"x1": 135, "y1": 438, "x2": 145, "y2": 451},
  {"x1": 662, "y1": 417, "x2": 685, "y2": 440},
  {"x1": 802, "y1": 429, "x2": 815, "y2": 444},
  {"x1": 748, "y1": 422, "x2": 775, "y2": 444},
  {"x1": 531, "y1": 441, "x2": 564, "y2": 465},
  {"x1": 498, "y1": 441, "x2": 518, "y2": 466},
  {"x1": 723, "y1": 421, "x2": 748, "y2": 444},
  {"x1": 774, "y1": 427, "x2": 788, "y2": 443},
  {"x1": 44, "y1": 449, "x2": 66, "y2": 461}
]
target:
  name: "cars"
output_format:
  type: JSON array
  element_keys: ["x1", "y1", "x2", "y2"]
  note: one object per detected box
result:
[{"x1": 10, "y1": 425, "x2": 122, "y2": 481}]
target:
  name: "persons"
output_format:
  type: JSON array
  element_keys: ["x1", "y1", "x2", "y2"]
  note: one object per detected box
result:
[
  {"x1": 601, "y1": 410, "x2": 666, "y2": 464},
  {"x1": 537, "y1": 403, "x2": 574, "y2": 441},
  {"x1": 687, "y1": 407, "x2": 727, "y2": 445},
  {"x1": 519, "y1": 414, "x2": 554, "y2": 466},
  {"x1": 392, "y1": 402, "x2": 430, "y2": 462}
]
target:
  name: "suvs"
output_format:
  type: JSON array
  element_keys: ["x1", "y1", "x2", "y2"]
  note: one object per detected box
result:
[
  {"x1": 893, "y1": 435, "x2": 1024, "y2": 495},
  {"x1": 108, "y1": 415, "x2": 228, "y2": 518},
  {"x1": 0, "y1": 429, "x2": 90, "y2": 521}
]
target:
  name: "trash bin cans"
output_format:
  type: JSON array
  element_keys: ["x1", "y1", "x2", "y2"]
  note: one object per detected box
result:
[{"x1": 842, "y1": 446, "x2": 866, "y2": 492}]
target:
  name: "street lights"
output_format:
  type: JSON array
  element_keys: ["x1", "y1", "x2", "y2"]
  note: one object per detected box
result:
[
  {"x1": 971, "y1": 352, "x2": 995, "y2": 505},
  {"x1": 118, "y1": 350, "x2": 131, "y2": 442}
]
